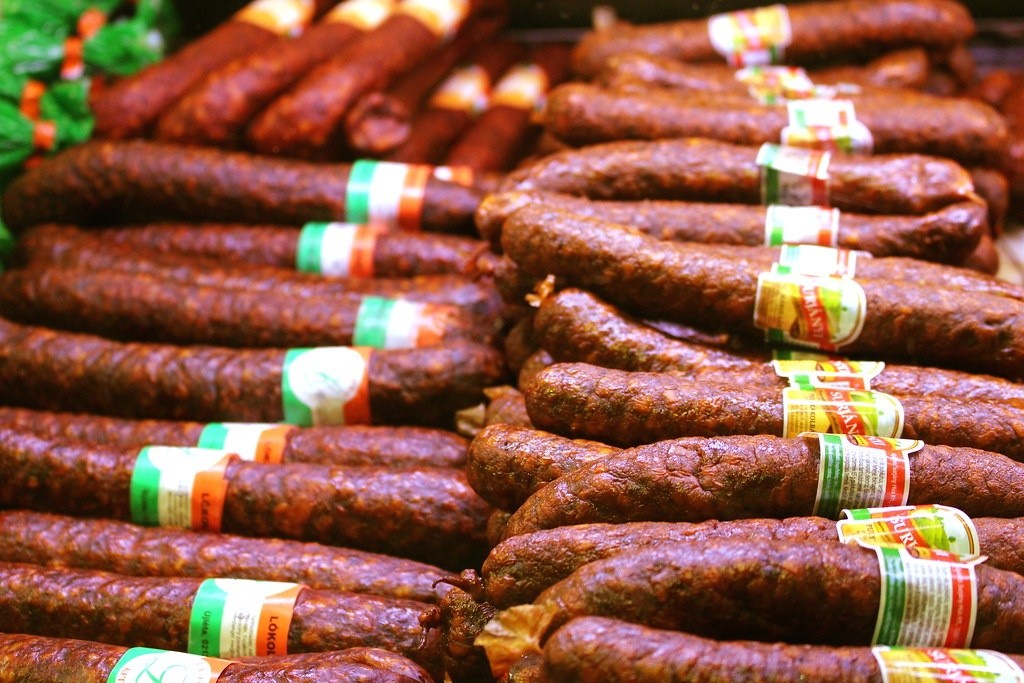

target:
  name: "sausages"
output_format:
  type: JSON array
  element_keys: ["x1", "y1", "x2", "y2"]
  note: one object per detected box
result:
[{"x1": 0, "y1": 0, "x2": 1024, "y2": 683}]
